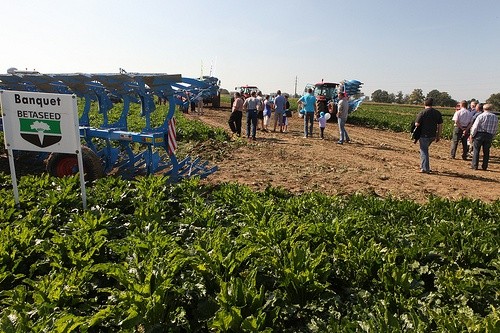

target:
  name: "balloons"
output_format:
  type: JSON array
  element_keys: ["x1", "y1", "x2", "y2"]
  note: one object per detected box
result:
[{"x1": 325, "y1": 113, "x2": 331, "y2": 120}]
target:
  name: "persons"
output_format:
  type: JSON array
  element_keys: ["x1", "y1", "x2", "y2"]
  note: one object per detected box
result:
[
  {"x1": 451, "y1": 100, "x2": 474, "y2": 160},
  {"x1": 297, "y1": 88, "x2": 318, "y2": 139},
  {"x1": 473, "y1": 103, "x2": 484, "y2": 124},
  {"x1": 467, "y1": 98, "x2": 479, "y2": 156},
  {"x1": 415, "y1": 97, "x2": 443, "y2": 173},
  {"x1": 271, "y1": 90, "x2": 286, "y2": 133},
  {"x1": 228, "y1": 92, "x2": 243, "y2": 137},
  {"x1": 255, "y1": 91, "x2": 266, "y2": 131},
  {"x1": 242, "y1": 92, "x2": 261, "y2": 140},
  {"x1": 315, "y1": 111, "x2": 326, "y2": 141},
  {"x1": 336, "y1": 92, "x2": 352, "y2": 144},
  {"x1": 261, "y1": 95, "x2": 275, "y2": 133},
  {"x1": 468, "y1": 103, "x2": 498, "y2": 171},
  {"x1": 157, "y1": 90, "x2": 205, "y2": 115},
  {"x1": 281, "y1": 95, "x2": 289, "y2": 133}
]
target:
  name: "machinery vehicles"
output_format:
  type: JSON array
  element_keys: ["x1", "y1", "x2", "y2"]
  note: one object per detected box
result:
[
  {"x1": 178, "y1": 75, "x2": 221, "y2": 111},
  {"x1": 230, "y1": 85, "x2": 289, "y2": 112},
  {"x1": 298, "y1": 82, "x2": 339, "y2": 123}
]
